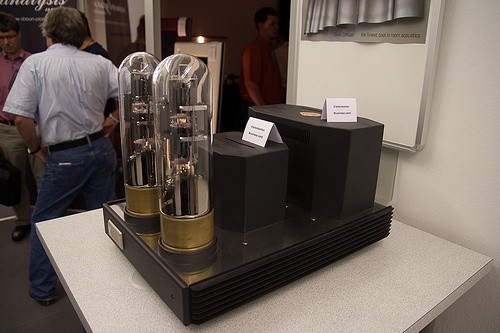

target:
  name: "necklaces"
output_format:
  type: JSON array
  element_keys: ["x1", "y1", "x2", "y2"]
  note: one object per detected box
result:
[{"x1": 86, "y1": 41, "x2": 95, "y2": 46}]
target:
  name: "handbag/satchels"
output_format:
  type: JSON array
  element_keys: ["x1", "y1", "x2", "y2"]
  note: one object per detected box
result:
[{"x1": 8, "y1": 69, "x2": 19, "y2": 90}]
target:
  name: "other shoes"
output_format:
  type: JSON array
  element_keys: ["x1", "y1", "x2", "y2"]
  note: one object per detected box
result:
[{"x1": 27, "y1": 290, "x2": 52, "y2": 305}]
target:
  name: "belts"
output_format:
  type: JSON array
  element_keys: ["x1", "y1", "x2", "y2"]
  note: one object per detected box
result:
[
  {"x1": 0, "y1": 119, "x2": 16, "y2": 126},
  {"x1": 47, "y1": 127, "x2": 104, "y2": 151}
]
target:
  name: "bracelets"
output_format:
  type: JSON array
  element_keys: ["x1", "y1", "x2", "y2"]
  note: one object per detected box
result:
[
  {"x1": 110, "y1": 113, "x2": 118, "y2": 123},
  {"x1": 30, "y1": 146, "x2": 41, "y2": 154}
]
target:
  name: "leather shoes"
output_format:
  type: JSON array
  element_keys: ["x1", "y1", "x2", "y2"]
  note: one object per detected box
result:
[{"x1": 10, "y1": 221, "x2": 29, "y2": 242}]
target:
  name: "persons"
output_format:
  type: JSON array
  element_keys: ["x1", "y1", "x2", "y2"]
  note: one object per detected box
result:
[
  {"x1": 0, "y1": 8, "x2": 118, "y2": 306},
  {"x1": 78, "y1": 10, "x2": 117, "y2": 118},
  {"x1": 119, "y1": 14, "x2": 146, "y2": 68},
  {"x1": 241, "y1": 7, "x2": 288, "y2": 104},
  {"x1": 0, "y1": 17, "x2": 46, "y2": 245}
]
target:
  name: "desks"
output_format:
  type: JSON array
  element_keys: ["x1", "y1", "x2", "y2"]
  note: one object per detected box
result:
[{"x1": 34, "y1": 206, "x2": 494, "y2": 333}]
